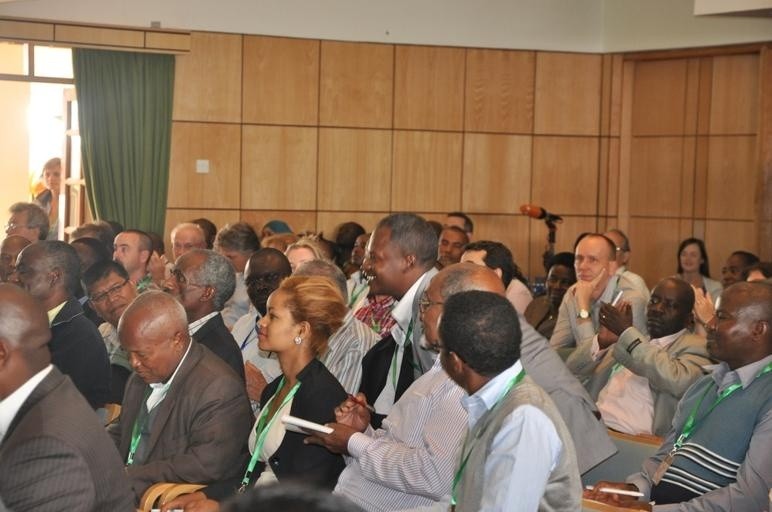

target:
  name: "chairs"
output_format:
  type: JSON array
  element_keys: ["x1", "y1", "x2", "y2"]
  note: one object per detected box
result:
[
  {"x1": 94, "y1": 400, "x2": 123, "y2": 427},
  {"x1": 157, "y1": 483, "x2": 207, "y2": 512},
  {"x1": 570, "y1": 431, "x2": 666, "y2": 511},
  {"x1": 138, "y1": 480, "x2": 174, "y2": 512}
]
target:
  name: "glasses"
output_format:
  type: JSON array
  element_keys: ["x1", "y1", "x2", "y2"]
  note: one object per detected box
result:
[{"x1": 88, "y1": 279, "x2": 130, "y2": 302}]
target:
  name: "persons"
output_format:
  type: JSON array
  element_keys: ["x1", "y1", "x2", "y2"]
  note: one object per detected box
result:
[
  {"x1": 32, "y1": 157, "x2": 61, "y2": 239},
  {"x1": 0, "y1": 203, "x2": 583, "y2": 512},
  {"x1": 550, "y1": 229, "x2": 772, "y2": 512}
]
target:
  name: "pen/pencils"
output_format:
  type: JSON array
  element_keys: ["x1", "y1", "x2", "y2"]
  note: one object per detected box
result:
[
  {"x1": 602, "y1": 290, "x2": 624, "y2": 318},
  {"x1": 585, "y1": 485, "x2": 644, "y2": 497},
  {"x1": 347, "y1": 393, "x2": 376, "y2": 413}
]
